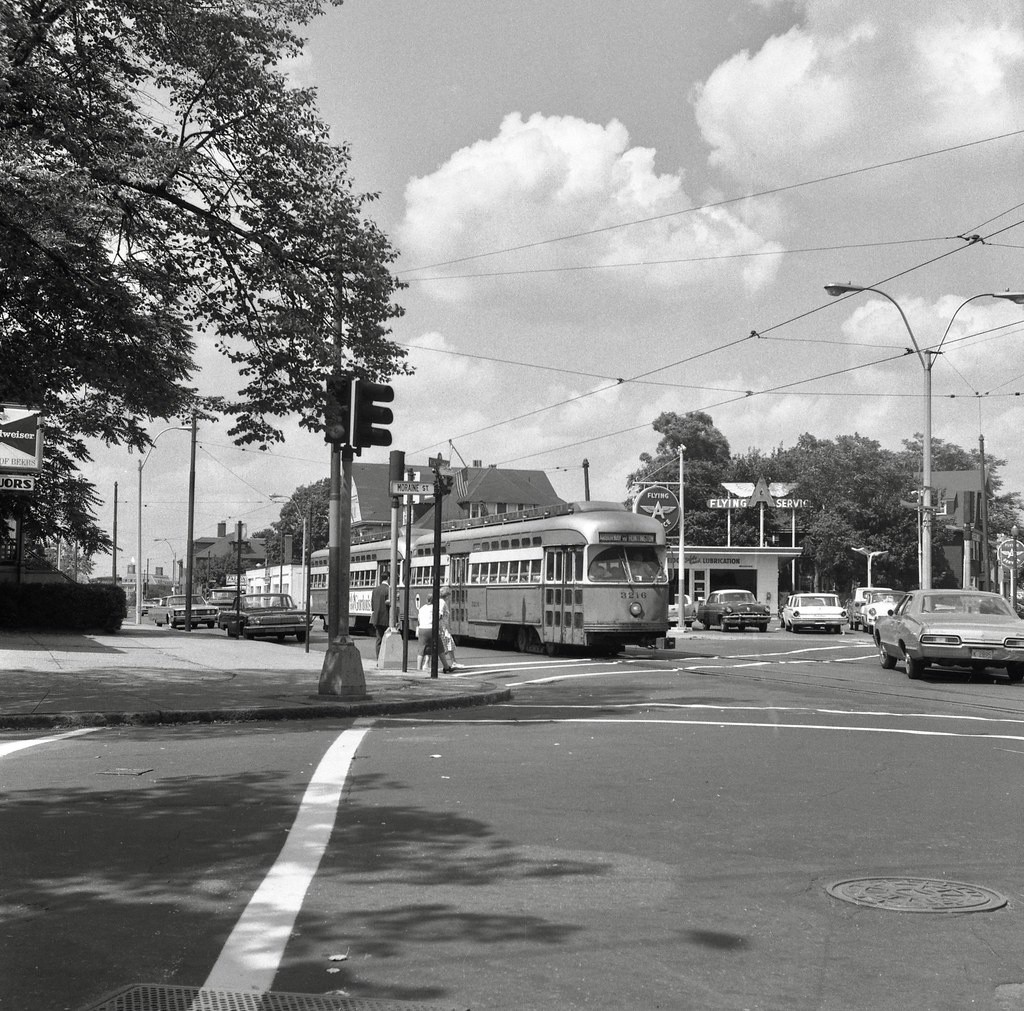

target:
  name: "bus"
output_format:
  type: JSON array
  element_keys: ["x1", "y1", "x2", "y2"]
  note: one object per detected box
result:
[{"x1": 309, "y1": 501, "x2": 675, "y2": 657}]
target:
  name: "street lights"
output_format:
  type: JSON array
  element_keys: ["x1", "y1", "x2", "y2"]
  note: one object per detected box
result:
[
  {"x1": 153, "y1": 539, "x2": 178, "y2": 604},
  {"x1": 134, "y1": 426, "x2": 201, "y2": 624},
  {"x1": 268, "y1": 493, "x2": 306, "y2": 611},
  {"x1": 821, "y1": 280, "x2": 1024, "y2": 613},
  {"x1": 850, "y1": 546, "x2": 888, "y2": 588},
  {"x1": 1010, "y1": 524, "x2": 1019, "y2": 612}
]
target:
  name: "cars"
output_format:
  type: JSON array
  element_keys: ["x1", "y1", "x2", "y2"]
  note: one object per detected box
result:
[
  {"x1": 860, "y1": 590, "x2": 906, "y2": 635},
  {"x1": 846, "y1": 586, "x2": 893, "y2": 632},
  {"x1": 667, "y1": 594, "x2": 697, "y2": 627},
  {"x1": 698, "y1": 590, "x2": 772, "y2": 633},
  {"x1": 218, "y1": 592, "x2": 314, "y2": 644},
  {"x1": 778, "y1": 592, "x2": 848, "y2": 634},
  {"x1": 141, "y1": 598, "x2": 164, "y2": 617},
  {"x1": 871, "y1": 588, "x2": 1024, "y2": 683}
]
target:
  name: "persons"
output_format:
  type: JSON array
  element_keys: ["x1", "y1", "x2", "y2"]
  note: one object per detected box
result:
[
  {"x1": 417, "y1": 593, "x2": 454, "y2": 674},
  {"x1": 423, "y1": 586, "x2": 465, "y2": 673},
  {"x1": 369, "y1": 572, "x2": 390, "y2": 668}
]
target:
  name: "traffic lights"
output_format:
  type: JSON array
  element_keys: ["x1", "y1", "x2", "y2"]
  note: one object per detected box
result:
[{"x1": 353, "y1": 378, "x2": 394, "y2": 449}]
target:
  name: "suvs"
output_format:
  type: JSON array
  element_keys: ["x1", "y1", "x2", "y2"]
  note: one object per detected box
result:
[{"x1": 207, "y1": 588, "x2": 247, "y2": 629}]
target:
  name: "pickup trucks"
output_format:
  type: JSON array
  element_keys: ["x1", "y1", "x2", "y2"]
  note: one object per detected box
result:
[{"x1": 147, "y1": 594, "x2": 220, "y2": 630}]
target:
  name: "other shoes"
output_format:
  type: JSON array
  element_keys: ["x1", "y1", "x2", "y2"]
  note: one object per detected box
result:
[
  {"x1": 443, "y1": 666, "x2": 455, "y2": 673},
  {"x1": 452, "y1": 663, "x2": 463, "y2": 668}
]
